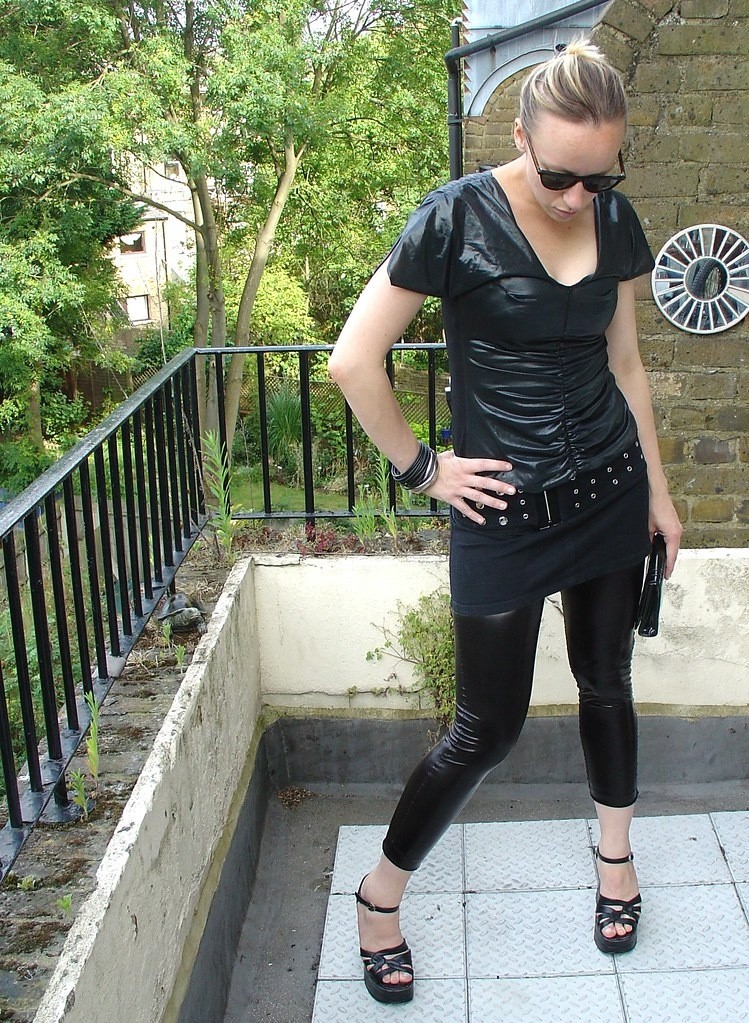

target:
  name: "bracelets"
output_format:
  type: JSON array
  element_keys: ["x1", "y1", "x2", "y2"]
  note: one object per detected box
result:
[{"x1": 391, "y1": 442, "x2": 440, "y2": 494}]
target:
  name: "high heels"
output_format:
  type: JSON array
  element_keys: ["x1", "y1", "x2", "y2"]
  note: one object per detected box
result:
[
  {"x1": 593, "y1": 843, "x2": 641, "y2": 952},
  {"x1": 355, "y1": 872, "x2": 414, "y2": 1003}
]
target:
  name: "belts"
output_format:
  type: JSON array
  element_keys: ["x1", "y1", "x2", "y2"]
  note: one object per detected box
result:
[{"x1": 452, "y1": 435, "x2": 648, "y2": 531}]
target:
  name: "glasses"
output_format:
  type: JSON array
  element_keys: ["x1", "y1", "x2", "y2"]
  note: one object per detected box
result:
[{"x1": 525, "y1": 133, "x2": 627, "y2": 193}]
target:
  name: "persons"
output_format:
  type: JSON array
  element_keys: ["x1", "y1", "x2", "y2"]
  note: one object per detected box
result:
[{"x1": 328, "y1": 41, "x2": 683, "y2": 1004}]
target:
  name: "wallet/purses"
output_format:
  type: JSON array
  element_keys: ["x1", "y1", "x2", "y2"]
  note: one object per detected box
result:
[{"x1": 634, "y1": 533, "x2": 667, "y2": 638}]
